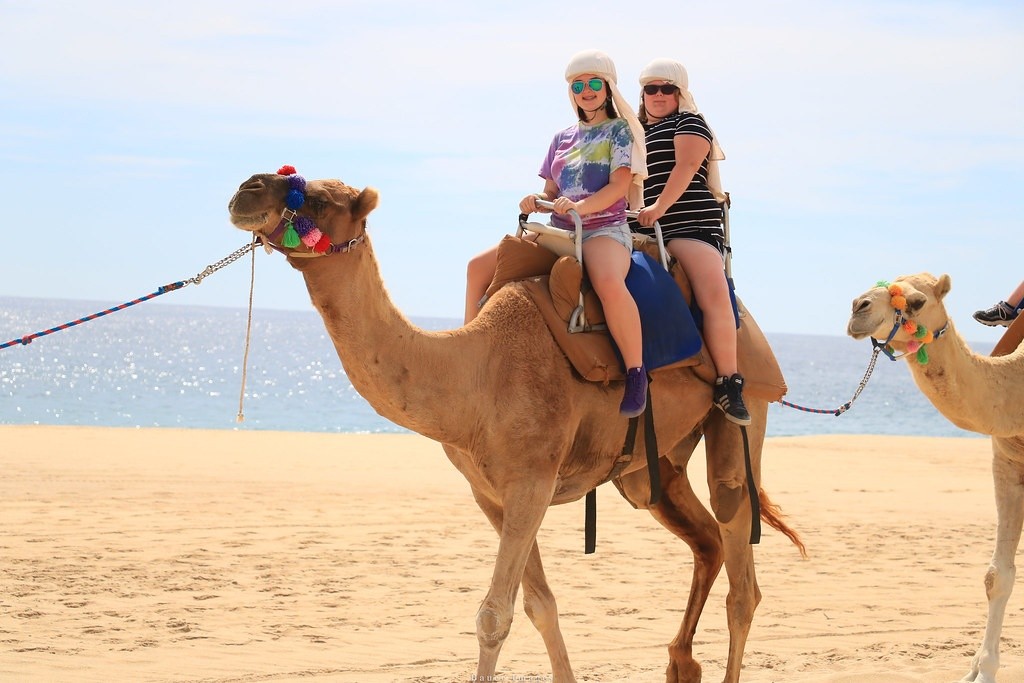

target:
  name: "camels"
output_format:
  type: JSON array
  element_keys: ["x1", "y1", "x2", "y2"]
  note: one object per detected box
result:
[
  {"x1": 846, "y1": 268, "x2": 1024, "y2": 682},
  {"x1": 228, "y1": 168, "x2": 809, "y2": 683}
]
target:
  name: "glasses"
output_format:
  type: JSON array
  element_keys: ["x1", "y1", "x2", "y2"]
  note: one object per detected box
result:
[
  {"x1": 569, "y1": 79, "x2": 603, "y2": 95},
  {"x1": 643, "y1": 84, "x2": 678, "y2": 95}
]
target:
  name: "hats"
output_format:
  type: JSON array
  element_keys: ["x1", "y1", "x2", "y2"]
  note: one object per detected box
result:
[
  {"x1": 639, "y1": 57, "x2": 726, "y2": 204},
  {"x1": 564, "y1": 50, "x2": 648, "y2": 213}
]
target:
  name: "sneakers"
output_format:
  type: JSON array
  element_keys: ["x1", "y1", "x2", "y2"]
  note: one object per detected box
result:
[
  {"x1": 713, "y1": 373, "x2": 752, "y2": 426},
  {"x1": 619, "y1": 363, "x2": 648, "y2": 419},
  {"x1": 972, "y1": 301, "x2": 1019, "y2": 327}
]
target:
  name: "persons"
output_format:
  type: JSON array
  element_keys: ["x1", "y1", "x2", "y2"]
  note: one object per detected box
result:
[
  {"x1": 625, "y1": 61, "x2": 753, "y2": 427},
  {"x1": 972, "y1": 280, "x2": 1024, "y2": 328},
  {"x1": 463, "y1": 52, "x2": 650, "y2": 419}
]
